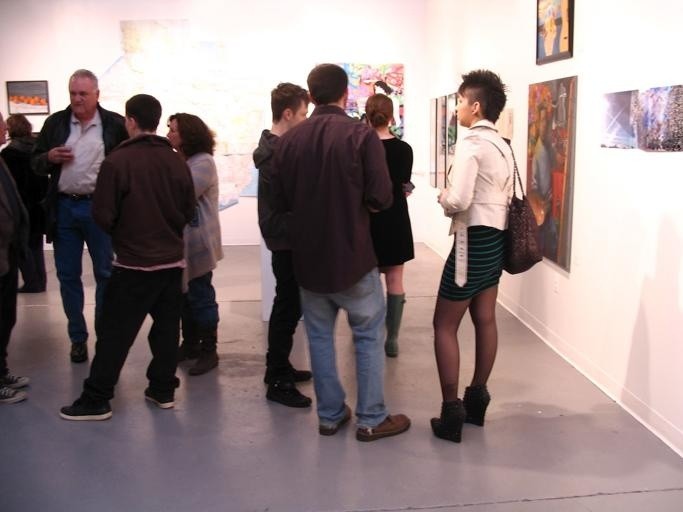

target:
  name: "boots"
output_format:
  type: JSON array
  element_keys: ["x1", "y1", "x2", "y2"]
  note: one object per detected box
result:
[{"x1": 384, "y1": 292, "x2": 406, "y2": 358}]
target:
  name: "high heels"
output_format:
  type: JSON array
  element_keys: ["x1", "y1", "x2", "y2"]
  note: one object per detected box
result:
[
  {"x1": 429, "y1": 398, "x2": 468, "y2": 444},
  {"x1": 461, "y1": 384, "x2": 491, "y2": 427}
]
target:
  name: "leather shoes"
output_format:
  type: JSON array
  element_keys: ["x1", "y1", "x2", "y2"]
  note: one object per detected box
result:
[
  {"x1": 318, "y1": 403, "x2": 352, "y2": 436},
  {"x1": 70, "y1": 340, "x2": 89, "y2": 364},
  {"x1": 17, "y1": 282, "x2": 46, "y2": 293},
  {"x1": 264, "y1": 367, "x2": 311, "y2": 383},
  {"x1": 266, "y1": 383, "x2": 312, "y2": 408},
  {"x1": 356, "y1": 413, "x2": 411, "y2": 441}
]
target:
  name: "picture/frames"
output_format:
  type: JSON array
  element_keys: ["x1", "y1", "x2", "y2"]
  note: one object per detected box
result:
[
  {"x1": 536, "y1": 0, "x2": 574, "y2": 65},
  {"x1": 5, "y1": 80, "x2": 50, "y2": 115}
]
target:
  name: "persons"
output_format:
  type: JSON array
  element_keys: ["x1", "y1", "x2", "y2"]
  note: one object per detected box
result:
[
  {"x1": 55, "y1": 93, "x2": 198, "y2": 424},
  {"x1": 0, "y1": 111, "x2": 53, "y2": 299},
  {"x1": 272, "y1": 63, "x2": 412, "y2": 443},
  {"x1": 424, "y1": 67, "x2": 513, "y2": 443},
  {"x1": 531, "y1": 105, "x2": 567, "y2": 260},
  {"x1": 0, "y1": 110, "x2": 32, "y2": 407},
  {"x1": 362, "y1": 96, "x2": 417, "y2": 359},
  {"x1": 249, "y1": 81, "x2": 313, "y2": 409},
  {"x1": 27, "y1": 66, "x2": 139, "y2": 362},
  {"x1": 166, "y1": 111, "x2": 224, "y2": 377}
]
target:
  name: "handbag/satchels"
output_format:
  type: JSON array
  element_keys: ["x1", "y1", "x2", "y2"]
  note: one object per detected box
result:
[{"x1": 501, "y1": 137, "x2": 544, "y2": 275}]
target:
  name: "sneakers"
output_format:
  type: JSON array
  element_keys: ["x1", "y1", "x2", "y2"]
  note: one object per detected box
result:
[
  {"x1": 178, "y1": 340, "x2": 219, "y2": 376},
  {"x1": 0, "y1": 386, "x2": 27, "y2": 404},
  {"x1": 58, "y1": 397, "x2": 113, "y2": 421},
  {"x1": 0, "y1": 371, "x2": 30, "y2": 389},
  {"x1": 144, "y1": 384, "x2": 176, "y2": 409}
]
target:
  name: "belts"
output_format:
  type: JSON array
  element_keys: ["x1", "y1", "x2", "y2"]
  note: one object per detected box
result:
[{"x1": 56, "y1": 192, "x2": 95, "y2": 202}]
